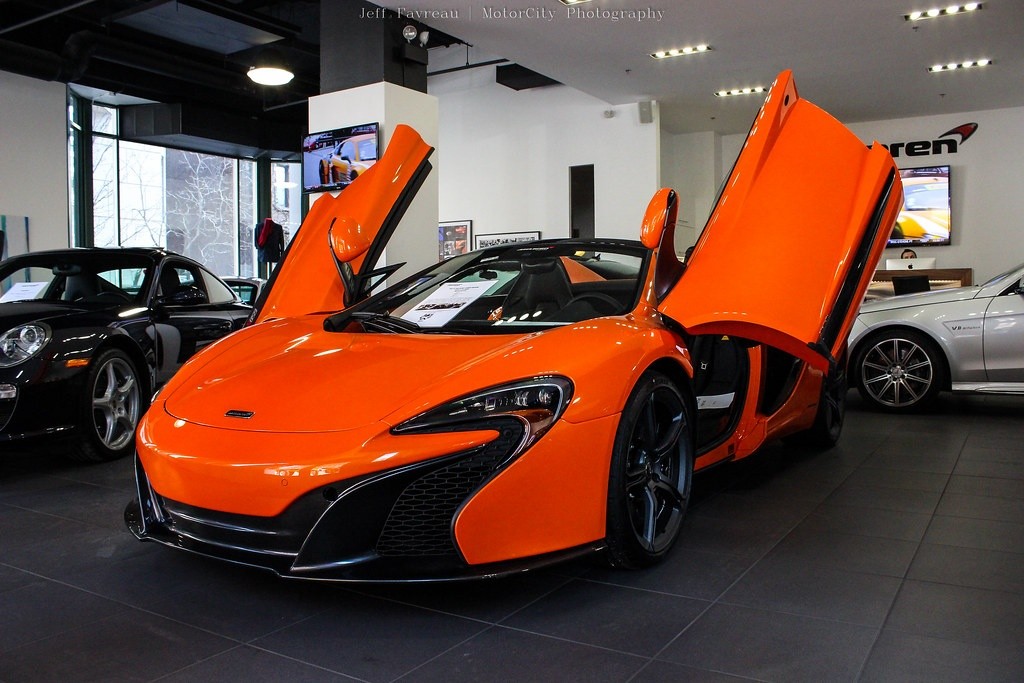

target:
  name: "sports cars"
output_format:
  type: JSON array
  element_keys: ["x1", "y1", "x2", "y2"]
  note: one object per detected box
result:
[{"x1": 125, "y1": 70, "x2": 904, "y2": 584}]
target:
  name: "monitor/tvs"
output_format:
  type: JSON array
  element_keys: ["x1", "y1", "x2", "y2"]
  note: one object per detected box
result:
[
  {"x1": 884, "y1": 165, "x2": 952, "y2": 248},
  {"x1": 300, "y1": 122, "x2": 380, "y2": 195},
  {"x1": 885, "y1": 258, "x2": 936, "y2": 269}
]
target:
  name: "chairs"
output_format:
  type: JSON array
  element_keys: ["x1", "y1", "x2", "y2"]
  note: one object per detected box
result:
[{"x1": 502, "y1": 257, "x2": 575, "y2": 325}]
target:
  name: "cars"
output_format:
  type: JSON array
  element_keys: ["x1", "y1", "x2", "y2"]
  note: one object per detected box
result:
[
  {"x1": 848, "y1": 264, "x2": 1024, "y2": 414},
  {"x1": 319, "y1": 133, "x2": 377, "y2": 185},
  {"x1": 0, "y1": 248, "x2": 267, "y2": 467},
  {"x1": 303, "y1": 136, "x2": 324, "y2": 151}
]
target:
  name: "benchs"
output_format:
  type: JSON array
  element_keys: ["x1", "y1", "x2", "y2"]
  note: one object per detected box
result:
[{"x1": 571, "y1": 279, "x2": 637, "y2": 314}]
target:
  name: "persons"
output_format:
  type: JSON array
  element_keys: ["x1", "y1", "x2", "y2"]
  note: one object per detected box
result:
[{"x1": 901, "y1": 248, "x2": 917, "y2": 259}]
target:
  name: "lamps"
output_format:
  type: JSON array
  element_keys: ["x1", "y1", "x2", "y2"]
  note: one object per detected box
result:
[
  {"x1": 246, "y1": 66, "x2": 295, "y2": 86},
  {"x1": 402, "y1": 25, "x2": 430, "y2": 65}
]
